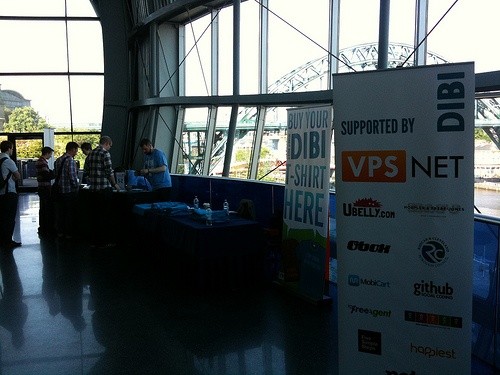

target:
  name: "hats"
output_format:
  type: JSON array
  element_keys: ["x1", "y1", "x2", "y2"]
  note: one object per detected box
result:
[{"x1": 41, "y1": 147, "x2": 54, "y2": 152}]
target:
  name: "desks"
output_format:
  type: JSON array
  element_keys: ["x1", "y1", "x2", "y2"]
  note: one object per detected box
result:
[
  {"x1": 132, "y1": 201, "x2": 261, "y2": 295},
  {"x1": 72, "y1": 188, "x2": 156, "y2": 241}
]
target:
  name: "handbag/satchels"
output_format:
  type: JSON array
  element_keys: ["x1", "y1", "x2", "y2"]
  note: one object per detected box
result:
[{"x1": 48, "y1": 182, "x2": 59, "y2": 203}]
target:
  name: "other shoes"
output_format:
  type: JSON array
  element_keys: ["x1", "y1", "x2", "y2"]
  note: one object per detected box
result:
[{"x1": 6, "y1": 240, "x2": 21, "y2": 249}]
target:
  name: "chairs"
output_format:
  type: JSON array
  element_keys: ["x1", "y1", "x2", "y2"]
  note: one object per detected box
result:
[{"x1": 236, "y1": 198, "x2": 257, "y2": 221}]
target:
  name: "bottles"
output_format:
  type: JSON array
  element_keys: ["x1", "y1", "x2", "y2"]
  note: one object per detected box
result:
[
  {"x1": 194, "y1": 196, "x2": 199, "y2": 209},
  {"x1": 223, "y1": 200, "x2": 229, "y2": 215},
  {"x1": 206, "y1": 205, "x2": 212, "y2": 226}
]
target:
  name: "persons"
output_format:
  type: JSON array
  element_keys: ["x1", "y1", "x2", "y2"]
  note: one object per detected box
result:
[
  {"x1": 53, "y1": 142, "x2": 80, "y2": 239},
  {"x1": 83, "y1": 136, "x2": 119, "y2": 248},
  {"x1": 136, "y1": 138, "x2": 172, "y2": 203},
  {"x1": 81, "y1": 142, "x2": 92, "y2": 156},
  {"x1": 0, "y1": 141, "x2": 22, "y2": 246},
  {"x1": 37, "y1": 147, "x2": 55, "y2": 235}
]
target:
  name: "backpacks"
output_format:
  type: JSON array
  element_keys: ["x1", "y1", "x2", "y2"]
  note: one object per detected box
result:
[{"x1": 0, "y1": 157, "x2": 12, "y2": 191}]
